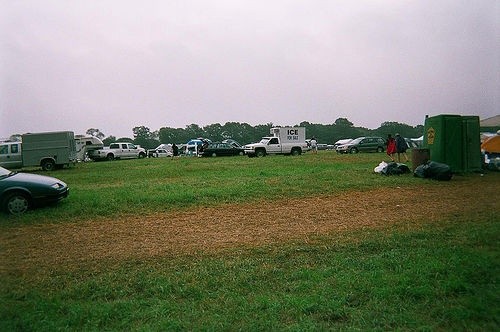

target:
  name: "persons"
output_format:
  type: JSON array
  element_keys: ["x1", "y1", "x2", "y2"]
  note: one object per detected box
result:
[
  {"x1": 170, "y1": 141, "x2": 208, "y2": 156},
  {"x1": 308, "y1": 137, "x2": 317, "y2": 152},
  {"x1": 385, "y1": 134, "x2": 396, "y2": 162},
  {"x1": 395, "y1": 133, "x2": 408, "y2": 162}
]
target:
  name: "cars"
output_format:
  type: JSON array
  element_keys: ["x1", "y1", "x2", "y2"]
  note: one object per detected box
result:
[
  {"x1": 0, "y1": 166, "x2": 70, "y2": 216},
  {"x1": 152, "y1": 138, "x2": 247, "y2": 158}
]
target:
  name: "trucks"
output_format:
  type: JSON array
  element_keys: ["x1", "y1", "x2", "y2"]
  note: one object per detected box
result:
[{"x1": 74, "y1": 134, "x2": 104, "y2": 163}]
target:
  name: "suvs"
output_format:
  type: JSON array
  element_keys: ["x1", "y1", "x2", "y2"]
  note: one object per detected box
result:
[{"x1": 336, "y1": 137, "x2": 387, "y2": 154}]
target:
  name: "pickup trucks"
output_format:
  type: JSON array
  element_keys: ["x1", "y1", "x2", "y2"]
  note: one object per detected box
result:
[
  {"x1": 86, "y1": 142, "x2": 147, "y2": 161},
  {"x1": 244, "y1": 126, "x2": 309, "y2": 157},
  {"x1": 0, "y1": 131, "x2": 78, "y2": 172}
]
